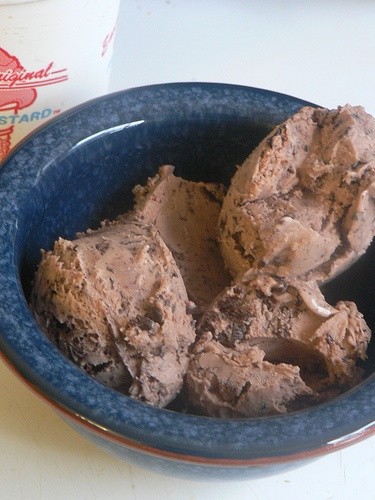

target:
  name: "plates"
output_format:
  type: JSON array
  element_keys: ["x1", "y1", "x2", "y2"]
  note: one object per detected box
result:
[{"x1": 0, "y1": 83, "x2": 375, "y2": 464}]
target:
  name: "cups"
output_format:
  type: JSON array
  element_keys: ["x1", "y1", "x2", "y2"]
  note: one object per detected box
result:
[{"x1": 0, "y1": 0, "x2": 119, "y2": 162}]
